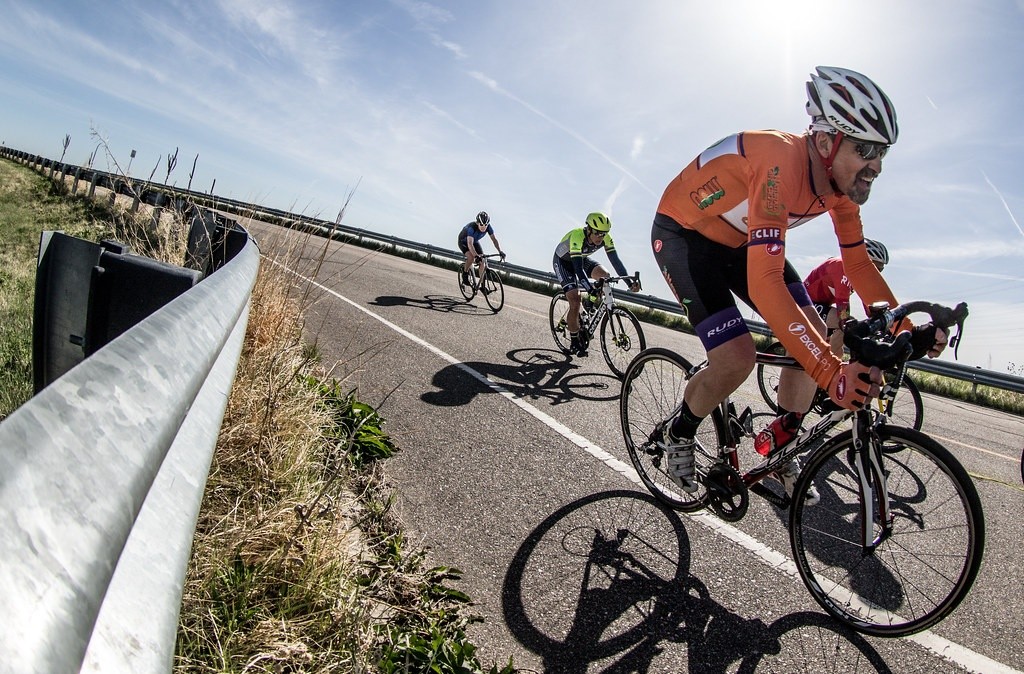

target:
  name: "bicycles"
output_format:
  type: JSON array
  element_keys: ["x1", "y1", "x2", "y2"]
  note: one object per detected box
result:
[
  {"x1": 757, "y1": 342, "x2": 923, "y2": 454},
  {"x1": 549, "y1": 271, "x2": 646, "y2": 380},
  {"x1": 457, "y1": 253, "x2": 507, "y2": 313},
  {"x1": 620, "y1": 302, "x2": 986, "y2": 639}
]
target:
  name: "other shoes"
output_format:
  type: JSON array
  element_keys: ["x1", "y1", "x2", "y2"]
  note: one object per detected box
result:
[
  {"x1": 480, "y1": 286, "x2": 489, "y2": 294},
  {"x1": 461, "y1": 267, "x2": 468, "y2": 282}
]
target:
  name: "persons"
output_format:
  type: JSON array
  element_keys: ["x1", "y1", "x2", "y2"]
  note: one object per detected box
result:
[
  {"x1": 458, "y1": 211, "x2": 506, "y2": 287},
  {"x1": 803, "y1": 237, "x2": 889, "y2": 354},
  {"x1": 651, "y1": 67, "x2": 950, "y2": 506},
  {"x1": 552, "y1": 212, "x2": 640, "y2": 358}
]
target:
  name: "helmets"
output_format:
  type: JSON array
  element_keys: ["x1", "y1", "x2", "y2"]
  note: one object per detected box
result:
[
  {"x1": 476, "y1": 211, "x2": 490, "y2": 225},
  {"x1": 806, "y1": 66, "x2": 899, "y2": 144},
  {"x1": 586, "y1": 211, "x2": 611, "y2": 232},
  {"x1": 864, "y1": 237, "x2": 889, "y2": 265}
]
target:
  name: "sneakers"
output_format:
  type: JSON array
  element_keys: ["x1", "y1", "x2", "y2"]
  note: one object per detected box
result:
[
  {"x1": 570, "y1": 343, "x2": 585, "y2": 357},
  {"x1": 582, "y1": 298, "x2": 595, "y2": 315},
  {"x1": 767, "y1": 455, "x2": 820, "y2": 505},
  {"x1": 663, "y1": 416, "x2": 698, "y2": 493}
]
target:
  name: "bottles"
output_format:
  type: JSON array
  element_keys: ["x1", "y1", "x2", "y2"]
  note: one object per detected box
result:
[
  {"x1": 580, "y1": 309, "x2": 596, "y2": 330},
  {"x1": 754, "y1": 411, "x2": 803, "y2": 456}
]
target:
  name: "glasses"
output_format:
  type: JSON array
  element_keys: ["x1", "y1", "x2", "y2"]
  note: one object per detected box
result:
[
  {"x1": 592, "y1": 228, "x2": 608, "y2": 238},
  {"x1": 844, "y1": 138, "x2": 890, "y2": 161},
  {"x1": 480, "y1": 223, "x2": 487, "y2": 226},
  {"x1": 877, "y1": 267, "x2": 884, "y2": 272}
]
